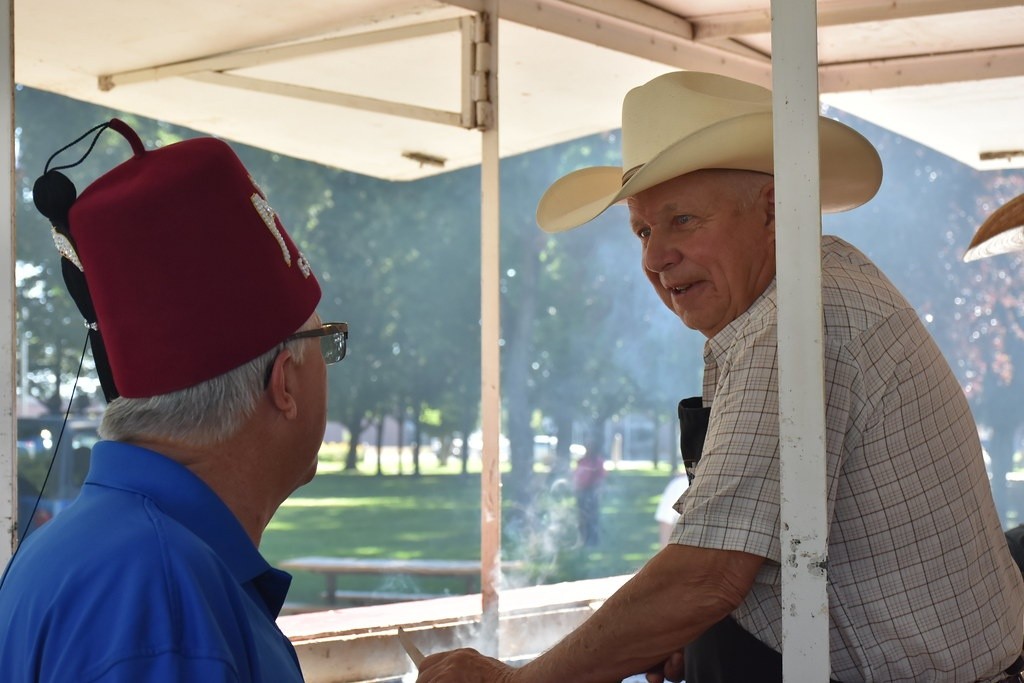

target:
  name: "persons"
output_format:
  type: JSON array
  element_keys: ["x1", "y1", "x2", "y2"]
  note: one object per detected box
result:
[
  {"x1": 656, "y1": 477, "x2": 691, "y2": 547},
  {"x1": 419, "y1": 71, "x2": 1024, "y2": 683},
  {"x1": 1, "y1": 136, "x2": 348, "y2": 683},
  {"x1": 577, "y1": 439, "x2": 603, "y2": 550}
]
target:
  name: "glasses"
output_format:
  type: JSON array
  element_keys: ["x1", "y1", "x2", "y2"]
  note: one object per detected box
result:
[{"x1": 264, "y1": 322, "x2": 349, "y2": 391}]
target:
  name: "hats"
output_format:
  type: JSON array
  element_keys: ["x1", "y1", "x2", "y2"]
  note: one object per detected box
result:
[
  {"x1": 962, "y1": 193, "x2": 1024, "y2": 262},
  {"x1": 535, "y1": 70, "x2": 883, "y2": 233},
  {"x1": 67, "y1": 118, "x2": 322, "y2": 397}
]
